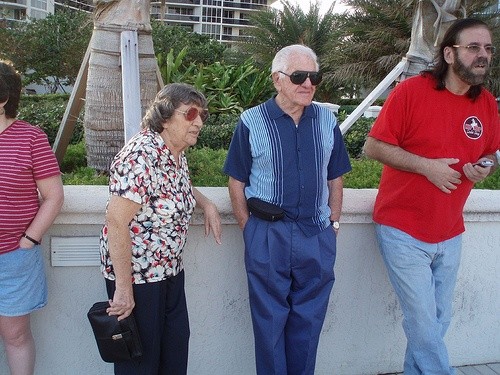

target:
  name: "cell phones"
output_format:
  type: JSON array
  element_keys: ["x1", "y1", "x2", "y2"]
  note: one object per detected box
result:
[{"x1": 472, "y1": 159, "x2": 494, "y2": 167}]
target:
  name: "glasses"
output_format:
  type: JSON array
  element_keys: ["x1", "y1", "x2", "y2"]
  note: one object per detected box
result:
[
  {"x1": 174, "y1": 105, "x2": 209, "y2": 122},
  {"x1": 278, "y1": 68, "x2": 322, "y2": 86},
  {"x1": 451, "y1": 43, "x2": 497, "y2": 56}
]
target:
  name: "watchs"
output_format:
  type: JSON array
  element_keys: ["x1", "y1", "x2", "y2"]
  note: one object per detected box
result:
[{"x1": 330, "y1": 220, "x2": 340, "y2": 230}]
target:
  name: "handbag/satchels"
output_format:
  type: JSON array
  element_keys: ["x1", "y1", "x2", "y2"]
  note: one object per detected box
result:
[{"x1": 86, "y1": 299, "x2": 144, "y2": 364}]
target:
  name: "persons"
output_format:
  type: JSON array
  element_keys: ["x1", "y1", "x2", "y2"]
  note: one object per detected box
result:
[
  {"x1": 98, "y1": 81, "x2": 224, "y2": 375},
  {"x1": 0, "y1": 59, "x2": 64, "y2": 375},
  {"x1": 362, "y1": 17, "x2": 500, "y2": 374},
  {"x1": 220, "y1": 44, "x2": 353, "y2": 375}
]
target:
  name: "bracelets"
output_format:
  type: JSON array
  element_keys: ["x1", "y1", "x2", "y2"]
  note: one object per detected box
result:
[{"x1": 22, "y1": 233, "x2": 40, "y2": 245}]
